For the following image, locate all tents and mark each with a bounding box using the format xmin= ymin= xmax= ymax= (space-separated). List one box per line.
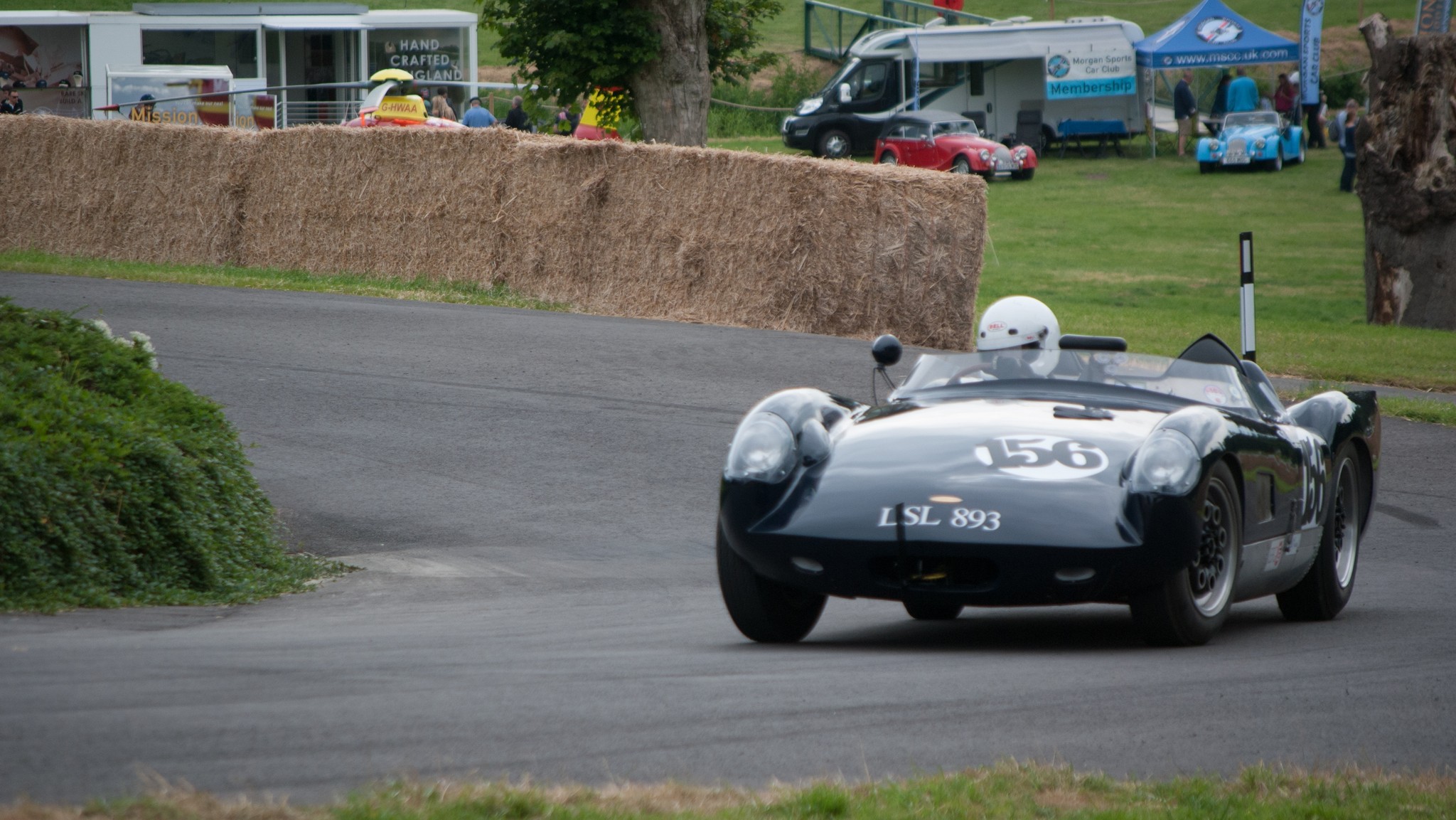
xmin=1131 ymin=0 xmax=1304 ymax=160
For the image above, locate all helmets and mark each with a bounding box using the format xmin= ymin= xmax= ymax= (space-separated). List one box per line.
xmin=976 ymin=296 xmax=1060 ymax=377
xmin=141 ymin=93 xmax=157 ymax=107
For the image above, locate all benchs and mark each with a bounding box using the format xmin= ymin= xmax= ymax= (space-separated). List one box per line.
xmin=1058 ymin=118 xmax=1129 ymax=159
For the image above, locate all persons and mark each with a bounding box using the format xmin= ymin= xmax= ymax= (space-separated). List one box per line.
xmin=418 ymin=87 xmax=591 ymax=136
xmin=1226 ymin=65 xmax=1261 ymax=113
xmin=1338 ymin=99 xmax=1359 ymax=156
xmin=1173 ymin=69 xmax=1197 ymax=159
xmin=0 ymin=84 xmax=23 ymax=115
xmin=1261 ymin=72 xmax=1294 ymax=120
xmin=1340 ymin=110 xmax=1360 ymax=195
xmin=128 ymin=93 xmax=162 ymax=123
xmin=970 ymin=295 xmax=1062 ymax=388
xmin=1216 ymin=73 xmax=1232 ymax=115
xmin=1288 ymin=67 xmax=1331 ymax=151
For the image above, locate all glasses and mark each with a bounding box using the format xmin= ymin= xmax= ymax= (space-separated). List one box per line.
xmin=9 ymin=95 xmax=18 ymax=99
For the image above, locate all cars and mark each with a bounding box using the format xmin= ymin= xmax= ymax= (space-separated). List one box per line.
xmin=875 ymin=109 xmax=1037 ymax=184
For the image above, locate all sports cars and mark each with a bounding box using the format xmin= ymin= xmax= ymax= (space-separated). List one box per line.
xmin=713 ymin=327 xmax=1382 ymax=652
xmin=1196 ymin=109 xmax=1307 ymax=173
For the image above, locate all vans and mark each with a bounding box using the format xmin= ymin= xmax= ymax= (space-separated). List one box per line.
xmin=775 ymin=13 xmax=1148 ymax=159
